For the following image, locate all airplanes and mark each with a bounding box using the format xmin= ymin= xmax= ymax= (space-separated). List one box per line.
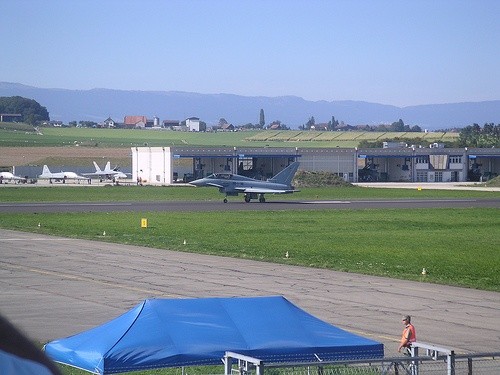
xmin=39 ymin=163 xmax=90 ymax=181
xmin=0 ymin=171 xmax=26 ymax=182
xmin=82 ymin=160 xmax=126 ymax=183
xmin=187 ymin=160 xmax=301 ymax=205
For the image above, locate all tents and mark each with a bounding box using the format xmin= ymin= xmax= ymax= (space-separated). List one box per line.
xmin=37 ymin=293 xmax=392 ymax=375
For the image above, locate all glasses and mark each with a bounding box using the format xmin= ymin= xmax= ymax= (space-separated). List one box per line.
xmin=402 ymin=319 xmax=408 ymax=321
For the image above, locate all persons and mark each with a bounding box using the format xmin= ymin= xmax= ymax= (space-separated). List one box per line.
xmin=397 ymin=315 xmax=416 ymax=358
